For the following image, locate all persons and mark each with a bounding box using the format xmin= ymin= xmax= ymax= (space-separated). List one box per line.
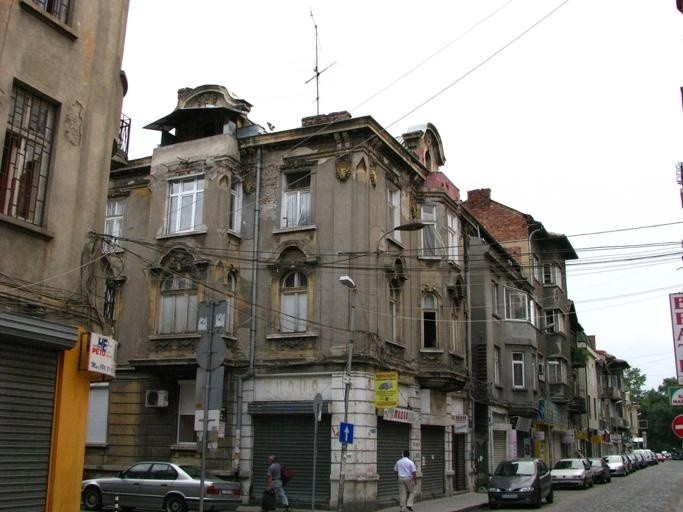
xmin=393 ymin=449 xmax=417 ymax=512
xmin=267 ymin=454 xmax=290 ymax=511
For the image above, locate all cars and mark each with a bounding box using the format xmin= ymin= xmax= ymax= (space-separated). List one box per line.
xmin=80 ymin=460 xmax=244 ymax=512
xmin=548 ymin=447 xmax=672 ymax=490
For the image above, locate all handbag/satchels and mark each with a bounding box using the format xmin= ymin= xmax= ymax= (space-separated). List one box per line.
xmin=281 ymin=467 xmax=294 ymax=486
xmin=262 ymin=489 xmax=277 ymax=511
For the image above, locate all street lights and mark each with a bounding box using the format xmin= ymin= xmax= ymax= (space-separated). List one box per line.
xmin=335 ymin=273 xmax=358 ymax=512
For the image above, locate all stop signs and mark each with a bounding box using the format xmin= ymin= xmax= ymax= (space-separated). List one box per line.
xmin=671 ymin=414 xmax=683 ymax=439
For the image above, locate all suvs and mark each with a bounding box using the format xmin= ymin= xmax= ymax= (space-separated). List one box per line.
xmin=487 ymin=456 xmax=557 ymax=509
